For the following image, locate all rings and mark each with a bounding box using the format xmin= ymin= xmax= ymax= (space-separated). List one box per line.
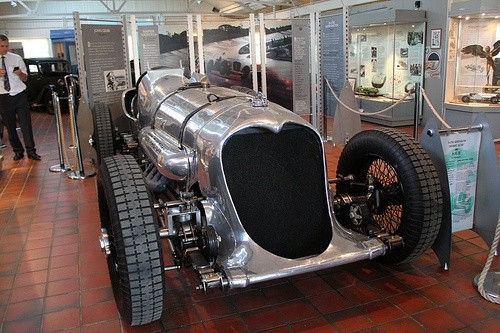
xmin=2 ymin=72 xmax=4 ymax=73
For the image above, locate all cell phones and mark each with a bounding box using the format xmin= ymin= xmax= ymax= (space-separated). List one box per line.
xmin=13 ymin=67 xmax=19 ymax=72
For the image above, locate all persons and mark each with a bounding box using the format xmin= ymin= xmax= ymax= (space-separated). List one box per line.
xmin=350 ymin=31 xmax=422 ymax=92
xmin=106 ymin=71 xmax=119 ymax=91
xmin=0 ymin=35 xmax=42 ymax=161
xmin=462 ymin=40 xmax=500 ymax=85
xmin=0 ymin=114 xmax=7 ymax=161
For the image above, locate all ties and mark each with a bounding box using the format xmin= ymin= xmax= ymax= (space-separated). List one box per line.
xmin=1 ymin=55 xmax=11 ymax=91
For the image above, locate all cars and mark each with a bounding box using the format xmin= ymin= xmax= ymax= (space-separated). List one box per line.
xmin=457 ymin=92 xmax=499 ymax=104
xmin=24 ymin=57 xmax=79 ymax=116
xmin=90 ymin=63 xmax=445 ymax=327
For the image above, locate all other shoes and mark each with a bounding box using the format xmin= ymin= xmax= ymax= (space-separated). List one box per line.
xmin=28 ymin=152 xmax=41 ymax=159
xmin=13 ymin=153 xmax=24 ymax=160
